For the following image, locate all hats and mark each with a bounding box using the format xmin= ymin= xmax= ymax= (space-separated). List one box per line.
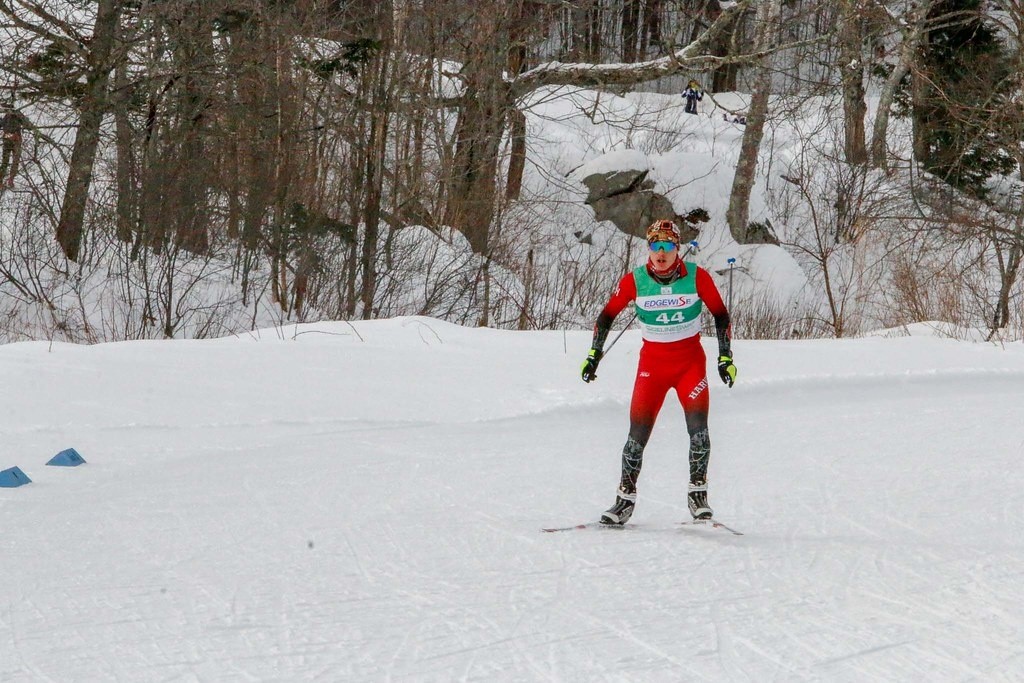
xmin=646 ymin=220 xmax=681 ymax=244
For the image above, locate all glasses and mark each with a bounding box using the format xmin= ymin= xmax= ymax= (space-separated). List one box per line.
xmin=651 ymin=241 xmax=674 ymax=251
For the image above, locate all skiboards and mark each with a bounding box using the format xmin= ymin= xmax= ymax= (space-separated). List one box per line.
xmin=540 ymin=517 xmax=744 ymax=537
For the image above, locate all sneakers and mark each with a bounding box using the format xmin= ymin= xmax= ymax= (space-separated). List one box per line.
xmin=601 ymin=495 xmax=635 ymax=525
xmin=688 ymin=489 xmax=713 ymax=519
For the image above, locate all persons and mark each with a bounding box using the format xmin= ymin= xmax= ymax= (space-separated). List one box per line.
xmin=682 ymin=79 xmax=704 ymax=114
xmin=0 ymin=104 xmax=33 ymax=187
xmin=580 ymin=220 xmax=737 ymax=527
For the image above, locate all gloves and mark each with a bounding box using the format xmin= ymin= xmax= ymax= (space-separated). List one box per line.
xmin=580 ymin=350 xmax=603 ymax=383
xmin=718 ymin=356 xmax=736 ymax=388
